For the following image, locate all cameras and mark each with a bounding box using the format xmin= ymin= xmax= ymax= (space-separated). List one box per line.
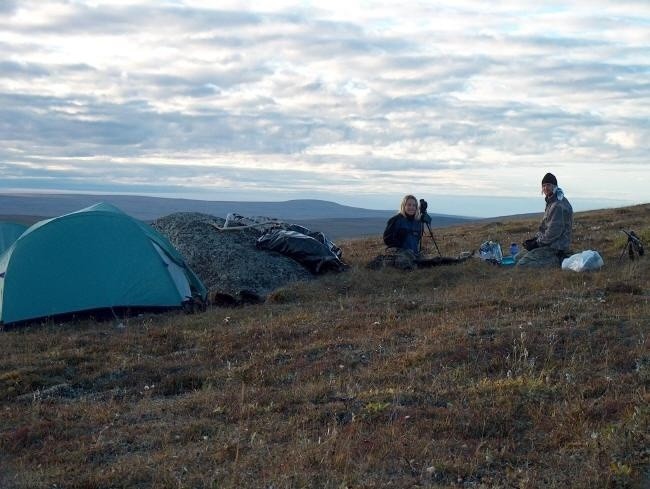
xmin=420 ymin=199 xmax=427 ymax=215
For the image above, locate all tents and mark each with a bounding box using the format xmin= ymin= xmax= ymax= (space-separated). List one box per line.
xmin=0 ymin=219 xmax=30 ymax=263
xmin=0 ymin=200 xmax=210 ymax=330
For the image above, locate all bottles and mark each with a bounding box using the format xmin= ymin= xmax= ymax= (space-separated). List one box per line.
xmin=498 ymin=257 xmax=518 ymax=265
xmin=510 ymin=243 xmax=518 ymax=258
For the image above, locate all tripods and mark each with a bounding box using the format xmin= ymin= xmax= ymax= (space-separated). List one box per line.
xmin=419 ymin=215 xmax=441 ymax=256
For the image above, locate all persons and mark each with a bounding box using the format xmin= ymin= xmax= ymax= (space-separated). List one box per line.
xmin=512 ymin=172 xmax=573 ymax=267
xmin=381 ymin=194 xmax=425 ymax=270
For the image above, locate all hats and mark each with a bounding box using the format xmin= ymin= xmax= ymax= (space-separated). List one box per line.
xmin=542 ymin=173 xmax=557 ymax=185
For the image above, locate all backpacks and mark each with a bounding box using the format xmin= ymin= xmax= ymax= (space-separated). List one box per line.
xmin=477 ymin=241 xmax=502 ymax=264
